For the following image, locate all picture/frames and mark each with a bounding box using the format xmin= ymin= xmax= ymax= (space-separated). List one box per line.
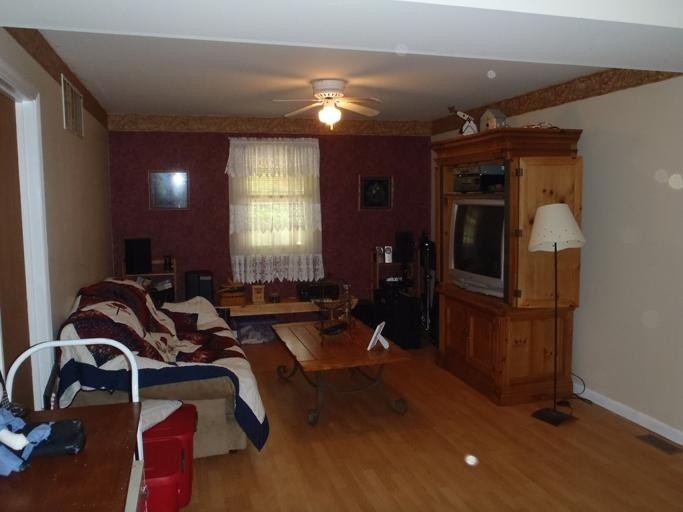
xmin=147 ymin=166 xmax=191 ymax=212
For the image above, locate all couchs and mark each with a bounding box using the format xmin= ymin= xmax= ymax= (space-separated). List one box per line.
xmin=55 ymin=276 xmax=252 ymax=459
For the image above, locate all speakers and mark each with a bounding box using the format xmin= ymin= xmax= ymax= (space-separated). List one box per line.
xmin=125 ymin=238 xmax=153 ymax=273
xmin=395 ymin=231 xmax=414 ymax=262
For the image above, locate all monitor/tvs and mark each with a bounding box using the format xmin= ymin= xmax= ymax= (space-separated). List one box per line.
xmin=448 ymin=198 xmax=505 ymax=299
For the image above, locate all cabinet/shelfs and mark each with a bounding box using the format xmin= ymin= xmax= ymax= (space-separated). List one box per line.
xmin=429 ymin=127 xmax=584 ymax=406
xmin=0 ymin=337 xmax=147 ymax=512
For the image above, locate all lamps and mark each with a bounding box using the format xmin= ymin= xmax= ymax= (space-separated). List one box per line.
xmin=318 ymin=98 xmax=341 ymax=125
xmin=527 ymin=203 xmax=589 ymax=426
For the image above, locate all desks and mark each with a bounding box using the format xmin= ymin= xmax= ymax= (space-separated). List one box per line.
xmin=229 ymin=293 xmax=358 ymax=346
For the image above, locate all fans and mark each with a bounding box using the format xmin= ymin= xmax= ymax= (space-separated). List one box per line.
xmin=270 ymin=79 xmax=384 ymax=119
